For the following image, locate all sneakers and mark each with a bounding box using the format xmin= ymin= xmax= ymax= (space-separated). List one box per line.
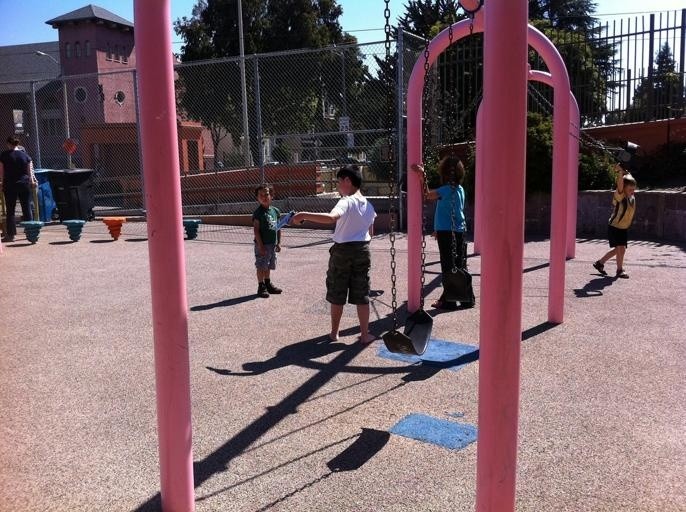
xmin=258 ymin=283 xmax=282 ymax=297
xmin=617 ymin=270 xmax=629 ymax=278
xmin=593 ymin=260 xmax=608 ymax=275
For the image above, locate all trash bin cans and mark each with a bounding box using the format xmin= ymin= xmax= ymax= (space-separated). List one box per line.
xmin=28 ymin=169 xmax=97 ymax=222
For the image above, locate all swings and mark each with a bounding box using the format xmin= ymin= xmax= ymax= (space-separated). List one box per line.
xmin=445 ymin=0 xmax=485 ymax=304
xmin=528 ymin=83 xmax=640 ymax=164
xmin=382 ymin=0 xmax=433 ymax=356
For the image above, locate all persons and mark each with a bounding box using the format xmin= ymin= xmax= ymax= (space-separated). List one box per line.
xmin=252 ymin=184 xmax=283 ymax=298
xmin=593 ymin=162 xmax=636 ymax=279
xmin=0 ymin=137 xmax=39 ymax=242
xmin=289 ymin=165 xmax=377 ymax=343
xmin=411 ymin=157 xmax=476 ymax=309
